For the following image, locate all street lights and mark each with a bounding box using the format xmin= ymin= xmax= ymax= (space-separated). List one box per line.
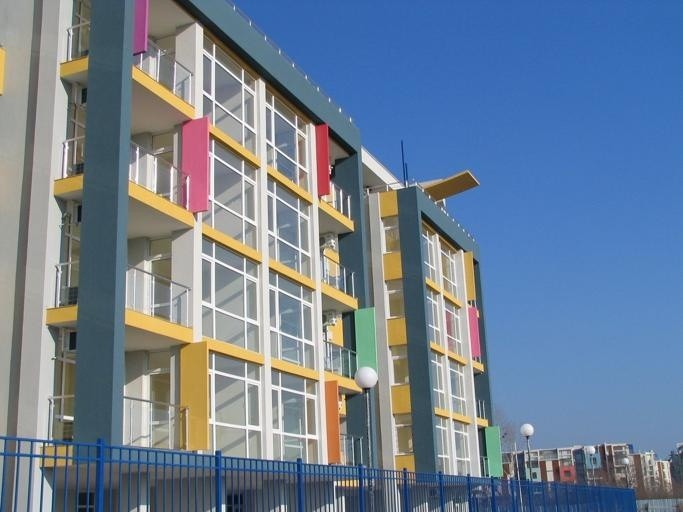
xmin=584 ymin=445 xmax=597 ymax=512
xmin=354 ymin=365 xmax=378 ymax=512
xmin=521 ymin=423 xmax=534 ymax=511
xmin=621 ymin=458 xmax=630 ymax=512
